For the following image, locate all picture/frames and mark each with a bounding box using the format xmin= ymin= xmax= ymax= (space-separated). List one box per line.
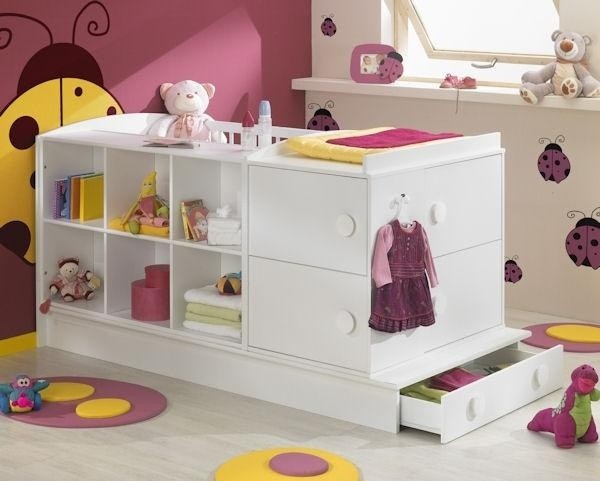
xmin=349 ymin=44 xmax=402 ymax=84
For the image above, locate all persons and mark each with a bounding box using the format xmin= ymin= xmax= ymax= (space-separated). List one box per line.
xmin=362 ymin=54 xmax=375 ymax=72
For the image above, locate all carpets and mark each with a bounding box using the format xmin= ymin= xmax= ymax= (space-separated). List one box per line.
xmin=522 ymin=322 xmax=599 ymax=353
xmin=217 ymin=448 xmax=362 ymax=481
xmin=2 ymin=377 xmax=168 ymax=429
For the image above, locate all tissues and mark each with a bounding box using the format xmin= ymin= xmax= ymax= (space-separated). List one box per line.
xmin=204 ymin=204 xmax=242 ymax=245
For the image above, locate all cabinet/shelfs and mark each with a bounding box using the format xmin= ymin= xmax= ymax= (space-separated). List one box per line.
xmin=375 ymin=148 xmax=506 ymax=261
xmin=370 ymin=240 xmax=507 ymax=371
xmin=248 ymin=161 xmax=372 ymax=273
xmin=34 ymin=113 xmax=248 ymax=393
xmin=368 ymin=328 xmax=565 ymax=443
xmin=249 ymin=256 xmax=370 ymax=421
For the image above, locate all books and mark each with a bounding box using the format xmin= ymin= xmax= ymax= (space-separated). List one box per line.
xmin=179 ymin=198 xmax=209 ymax=245
xmin=49 ymin=169 xmax=104 ymax=224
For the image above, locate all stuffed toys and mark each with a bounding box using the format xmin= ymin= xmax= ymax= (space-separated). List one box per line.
xmin=526 ymin=362 xmax=599 ymax=451
xmin=37 ymin=256 xmax=101 ymax=316
xmin=152 ymin=80 xmax=227 ymax=143
xmin=519 ymin=28 xmax=599 ymax=106
xmin=1 ymin=374 xmax=50 ymax=417
xmin=120 ymin=168 xmax=171 ymax=236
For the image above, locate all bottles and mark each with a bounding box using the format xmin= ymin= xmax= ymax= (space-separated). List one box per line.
xmin=241 ymin=99 xmax=273 ymax=151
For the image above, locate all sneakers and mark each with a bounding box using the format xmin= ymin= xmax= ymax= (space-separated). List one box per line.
xmin=458 ymin=77 xmax=476 ymax=89
xmin=439 ymin=73 xmax=458 ymax=88
